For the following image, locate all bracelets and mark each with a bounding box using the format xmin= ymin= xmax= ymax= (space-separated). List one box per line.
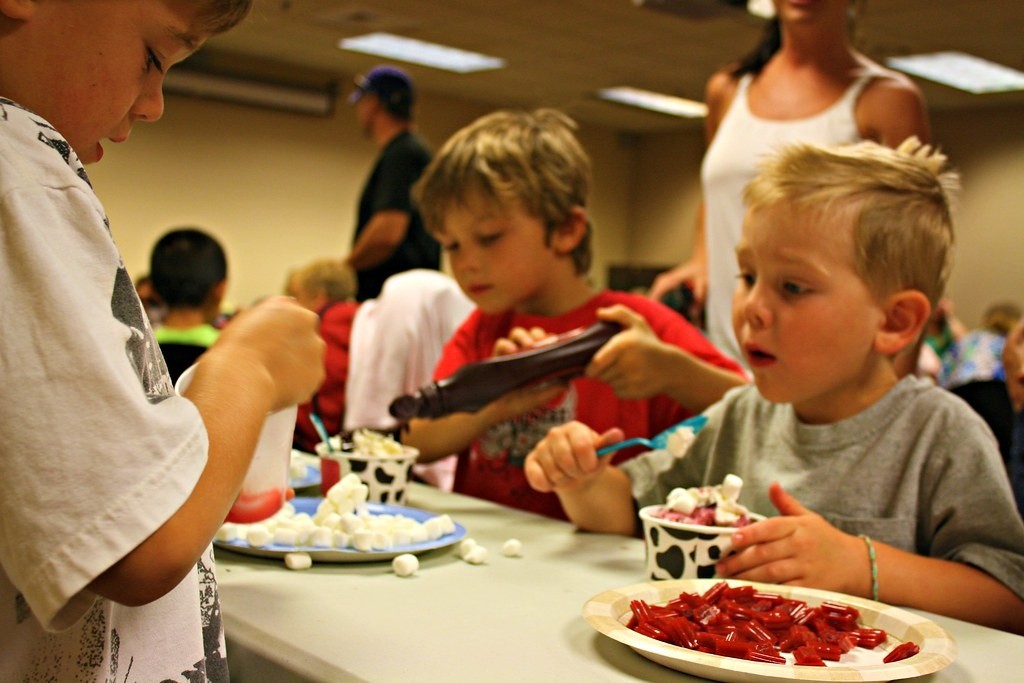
xmin=857 ymin=534 xmax=878 ymax=601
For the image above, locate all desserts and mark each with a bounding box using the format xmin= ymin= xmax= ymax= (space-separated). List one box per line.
xmin=493 ymin=326 xmax=546 ymax=355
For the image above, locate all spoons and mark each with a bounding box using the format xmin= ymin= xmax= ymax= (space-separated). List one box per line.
xmin=596 ymin=414 xmax=708 ymax=455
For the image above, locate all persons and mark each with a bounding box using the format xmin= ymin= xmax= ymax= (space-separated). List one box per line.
xmin=527 ymin=137 xmax=1024 ymax=635
xmin=650 ymin=0 xmax=929 ymax=377
xmin=334 ymin=68 xmax=445 ymax=303
xmin=279 ymin=267 xmax=361 ymax=454
xmin=400 ymin=107 xmax=745 ymax=520
xmin=143 ymin=227 xmax=229 ymax=393
xmin=1 ymin=0 xmax=325 ymax=683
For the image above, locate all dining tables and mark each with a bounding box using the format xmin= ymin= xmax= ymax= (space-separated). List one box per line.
xmin=214 ymin=477 xmax=1024 ymax=681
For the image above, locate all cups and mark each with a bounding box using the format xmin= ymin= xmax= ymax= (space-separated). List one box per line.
xmin=174 ymin=360 xmax=297 ymax=524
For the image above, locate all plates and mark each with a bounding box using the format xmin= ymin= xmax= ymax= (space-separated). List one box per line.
xmin=212 ymin=497 xmax=467 ymax=563
xmin=289 ymin=465 xmax=321 ymax=494
xmin=580 ymin=578 xmax=957 ymax=682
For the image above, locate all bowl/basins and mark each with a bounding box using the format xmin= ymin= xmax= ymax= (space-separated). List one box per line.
xmin=314 ymin=442 xmax=419 ymax=505
xmin=639 ymin=505 xmax=768 ymax=580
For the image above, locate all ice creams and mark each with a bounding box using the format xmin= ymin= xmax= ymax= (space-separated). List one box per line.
xmin=327 ymin=428 xmax=401 ymax=456
xmin=648 ymin=473 xmax=761 ymax=526
